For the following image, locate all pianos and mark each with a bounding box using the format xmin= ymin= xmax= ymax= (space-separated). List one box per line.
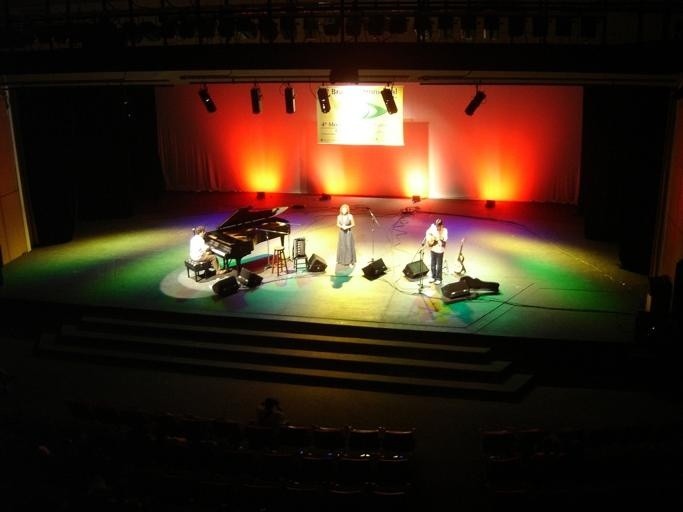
xmin=202 ymin=206 xmax=290 ymax=277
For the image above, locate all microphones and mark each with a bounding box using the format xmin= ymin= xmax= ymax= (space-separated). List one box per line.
xmin=421 ymin=237 xmax=426 ymax=246
xmin=368 ymin=208 xmax=373 ymax=216
xmin=191 ymin=227 xmax=195 ymax=235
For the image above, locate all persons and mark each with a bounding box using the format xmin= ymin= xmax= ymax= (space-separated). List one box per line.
xmin=255 ymin=397 xmax=287 ymax=427
xmin=188 ymin=226 xmax=226 ymax=276
xmin=425 ymin=218 xmax=448 ymax=285
xmin=335 ymin=204 xmax=355 ymax=266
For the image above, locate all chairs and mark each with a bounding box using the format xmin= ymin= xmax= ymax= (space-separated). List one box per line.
xmin=293 ymin=244 xmax=309 ymax=272
xmin=169 ymin=411 xmax=425 ymax=464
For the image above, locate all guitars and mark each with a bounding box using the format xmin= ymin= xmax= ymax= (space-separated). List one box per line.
xmin=428 ymin=233 xmax=444 ymax=247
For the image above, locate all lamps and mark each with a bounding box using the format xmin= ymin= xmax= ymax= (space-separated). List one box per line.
xmin=193 ymin=76 xmax=488 ymax=117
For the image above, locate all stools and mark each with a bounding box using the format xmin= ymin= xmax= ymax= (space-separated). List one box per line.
xmin=272 ymin=247 xmax=289 ymax=275
xmin=185 ymin=257 xmax=220 ymax=283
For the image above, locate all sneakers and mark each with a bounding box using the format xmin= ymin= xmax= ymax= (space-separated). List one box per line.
xmin=428 ymin=278 xmax=441 ymax=285
xmin=215 ymin=270 xmax=227 ymax=275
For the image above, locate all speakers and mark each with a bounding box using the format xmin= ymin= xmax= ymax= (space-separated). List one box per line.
xmin=441 ymin=280 xmax=470 ymax=298
xmin=308 ymin=253 xmax=327 ymax=272
xmin=212 ymin=276 xmax=240 ymax=295
xmin=361 ymin=257 xmax=388 ymax=277
xmin=237 ymin=266 xmax=263 ymax=286
xmin=402 ymin=260 xmax=429 ymax=279
